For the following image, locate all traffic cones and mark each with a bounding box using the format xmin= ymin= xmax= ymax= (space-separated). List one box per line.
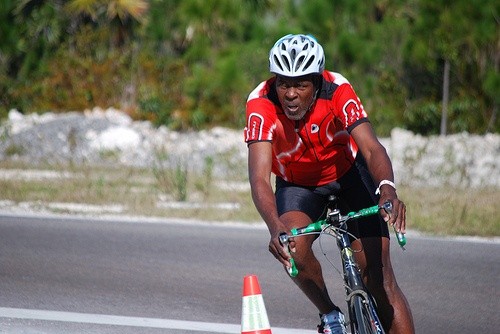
xmin=241 ymin=274 xmax=273 ymax=334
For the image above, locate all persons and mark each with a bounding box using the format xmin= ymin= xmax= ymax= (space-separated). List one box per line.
xmin=245 ymin=33 xmax=415 ymax=333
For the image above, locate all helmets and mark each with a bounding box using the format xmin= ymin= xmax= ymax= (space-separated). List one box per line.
xmin=269 ymin=33 xmax=326 ymax=77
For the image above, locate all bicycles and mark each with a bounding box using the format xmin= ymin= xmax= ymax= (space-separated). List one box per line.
xmin=277 ymin=196 xmax=407 ymax=334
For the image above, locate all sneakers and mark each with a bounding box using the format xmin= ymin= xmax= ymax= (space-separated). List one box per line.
xmin=321 ymin=307 xmax=347 ymax=334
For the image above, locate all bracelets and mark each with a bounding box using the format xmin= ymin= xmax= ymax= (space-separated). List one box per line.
xmin=375 ymin=179 xmax=396 ymax=194
